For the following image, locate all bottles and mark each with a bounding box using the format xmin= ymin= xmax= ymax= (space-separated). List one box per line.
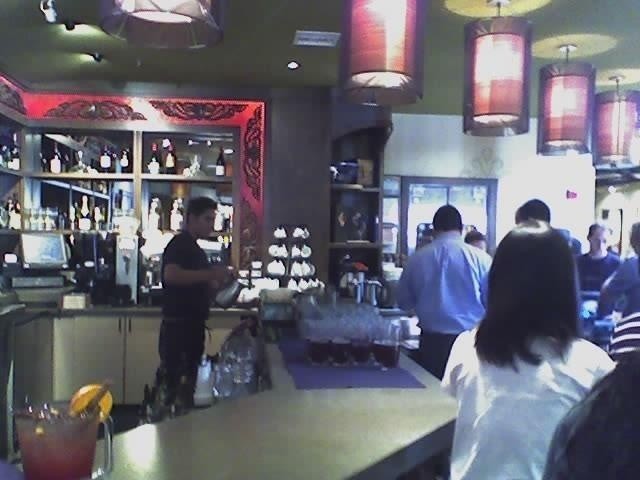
xmin=0 ymin=142 xmax=230 ymax=231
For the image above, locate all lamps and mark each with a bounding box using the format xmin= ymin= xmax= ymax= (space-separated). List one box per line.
xmin=335 ymin=0 xmax=423 ymax=107
xmin=96 ymin=0 xmax=223 ymax=51
xmin=460 ymin=0 xmax=640 ymax=169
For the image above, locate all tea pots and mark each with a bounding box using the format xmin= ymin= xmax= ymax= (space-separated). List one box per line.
xmin=215 ymin=279 xmax=252 ymax=311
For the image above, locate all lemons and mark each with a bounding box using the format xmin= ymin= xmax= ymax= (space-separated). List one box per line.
xmin=69 ymin=383 xmax=114 ymax=422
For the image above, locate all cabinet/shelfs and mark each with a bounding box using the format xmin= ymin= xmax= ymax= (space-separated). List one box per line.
xmin=327 ymin=86 xmax=393 ymax=289
xmin=8 ymin=315 xmax=246 ymax=409
xmin=0 ymin=71 xmax=266 ymax=303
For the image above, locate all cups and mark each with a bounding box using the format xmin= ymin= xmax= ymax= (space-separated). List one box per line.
xmin=14 ymin=398 xmax=116 ymax=480
xmin=298 ymin=314 xmax=401 ymax=371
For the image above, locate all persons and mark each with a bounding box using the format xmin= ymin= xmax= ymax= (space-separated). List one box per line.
xmin=540 ymin=351 xmax=640 ymax=479
xmin=515 ymin=201 xmax=584 ymax=257
xmin=606 ymin=283 xmax=640 ymax=368
xmin=594 ymin=220 xmax=640 ymax=317
xmin=462 ymin=230 xmax=487 ymax=252
xmin=419 ymin=229 xmax=434 ymax=245
xmin=572 ymin=221 xmax=625 ymax=352
xmin=396 ymin=203 xmax=494 ymax=382
xmin=441 ymin=218 xmax=616 ymax=479
xmin=156 ymin=195 xmax=244 ymax=420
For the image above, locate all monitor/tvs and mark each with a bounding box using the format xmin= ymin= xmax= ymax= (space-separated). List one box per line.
xmin=20 ymin=231 xmax=70 ymax=276
